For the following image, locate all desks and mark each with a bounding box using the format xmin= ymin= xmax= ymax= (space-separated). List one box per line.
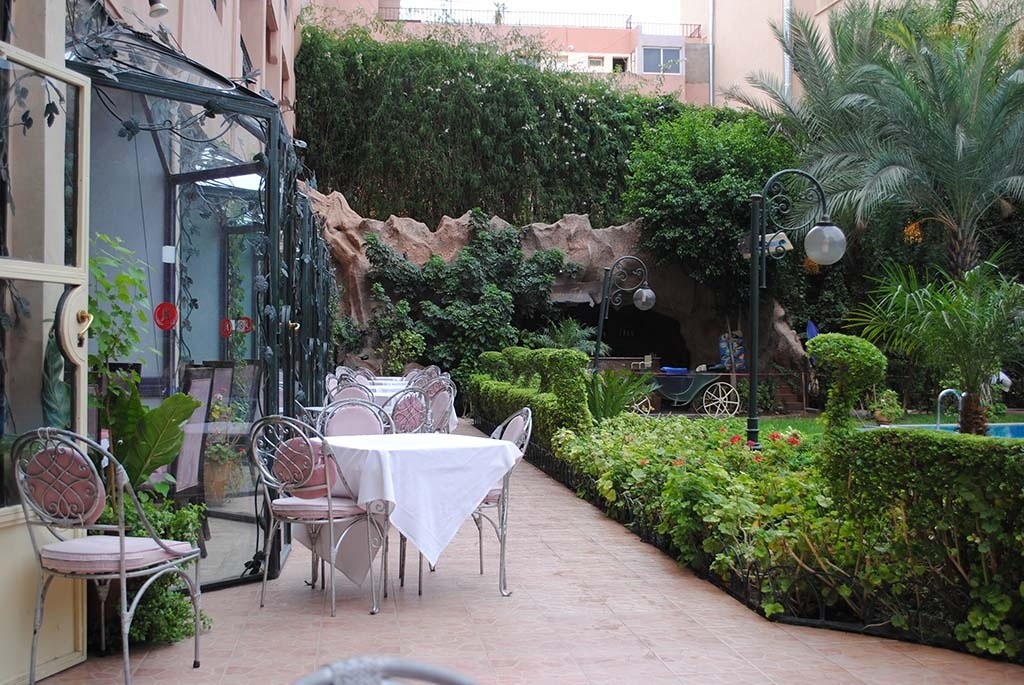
xmin=302 ymin=406 xmax=394 ymax=428
xmin=306 ymin=434 xmax=521 ymax=614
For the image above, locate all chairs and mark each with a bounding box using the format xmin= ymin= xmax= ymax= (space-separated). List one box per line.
xmin=10 ymin=427 xmax=202 ymax=685
xmin=248 ymin=362 xmax=532 ymax=619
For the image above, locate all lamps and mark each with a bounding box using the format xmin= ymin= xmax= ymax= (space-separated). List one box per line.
xmin=147 ymin=0 xmax=168 ymax=18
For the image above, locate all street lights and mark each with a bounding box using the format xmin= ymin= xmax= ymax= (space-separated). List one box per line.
xmin=747 ymin=169 xmax=847 ymax=450
xmin=593 ymin=256 xmax=657 ymax=369
